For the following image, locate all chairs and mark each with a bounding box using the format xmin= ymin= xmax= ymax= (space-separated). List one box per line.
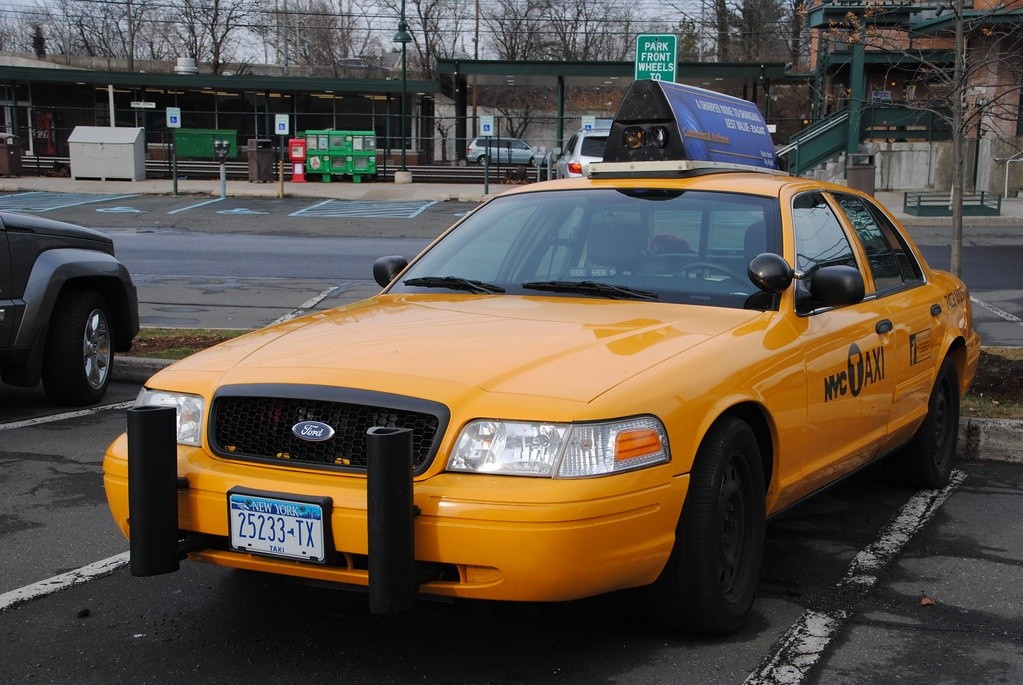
xmin=722 ymin=216 xmax=804 ymax=294
xmin=568 ymin=220 xmax=647 ymax=288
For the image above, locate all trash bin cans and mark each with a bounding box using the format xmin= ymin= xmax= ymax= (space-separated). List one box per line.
xmin=33 ymin=138 xmax=48 ymax=156
xmin=0 ymin=133 xmax=23 ymax=177
xmin=247 ymin=139 xmax=273 ymax=182
xmin=845 ymin=151 xmax=876 ymax=196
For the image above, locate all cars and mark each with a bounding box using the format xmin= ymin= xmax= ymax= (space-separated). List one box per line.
xmin=553 ymin=126 xmax=610 ymax=180
xmin=103 ymin=80 xmax=982 ymax=646
xmin=1 ymin=211 xmax=141 ymax=409
xmin=465 ymin=138 xmax=536 ymax=168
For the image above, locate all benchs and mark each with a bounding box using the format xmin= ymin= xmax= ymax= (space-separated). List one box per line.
xmin=650 ymin=254 xmax=746 ymax=290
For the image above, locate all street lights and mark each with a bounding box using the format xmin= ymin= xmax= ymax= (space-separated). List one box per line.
xmin=394 ymin=0 xmax=416 ymax=183
